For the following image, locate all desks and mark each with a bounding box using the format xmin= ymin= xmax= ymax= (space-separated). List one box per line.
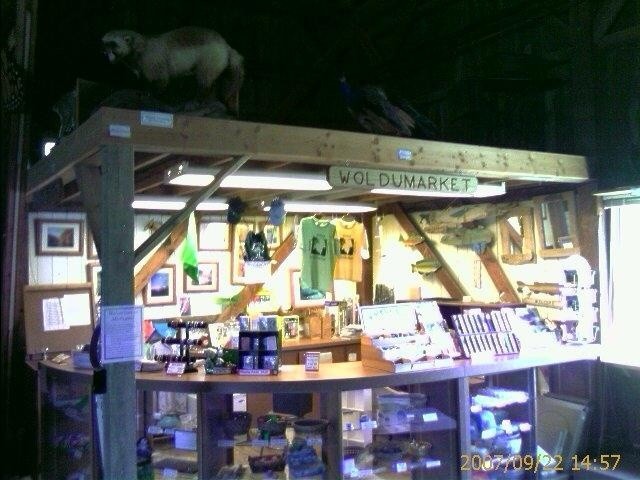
xmin=396 ymin=298 xmax=526 ymax=330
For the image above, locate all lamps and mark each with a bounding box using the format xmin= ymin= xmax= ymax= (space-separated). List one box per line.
xmin=369 ymin=180 xmax=507 ymax=199
xmin=163 ymin=164 xmax=334 ymax=192
xmin=133 ymin=191 xmax=232 ymax=215
xmin=257 ymin=197 xmax=380 ymax=215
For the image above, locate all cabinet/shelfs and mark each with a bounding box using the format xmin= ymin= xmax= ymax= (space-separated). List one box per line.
xmin=38 ymin=333 xmax=599 ymax=480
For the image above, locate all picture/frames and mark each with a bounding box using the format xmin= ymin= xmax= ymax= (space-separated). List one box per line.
xmin=231 ymin=221 xmax=256 ymax=287
xmin=288 ymin=266 xmax=327 ymax=310
xmin=139 ymin=262 xmax=178 ymax=308
xmin=87 ymin=227 xmax=100 ymax=261
xmin=86 ymin=261 xmax=104 ymax=332
xmin=195 ymin=217 xmax=233 ymax=252
xmin=181 ymin=260 xmax=220 ymax=294
xmin=258 ymin=220 xmax=282 ymax=252
xmin=36 ymin=215 xmax=86 ymax=258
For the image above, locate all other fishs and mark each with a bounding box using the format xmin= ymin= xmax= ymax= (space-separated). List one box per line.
xmin=411 ymin=259 xmax=442 ymax=277
xmin=398 ymin=233 xmax=426 ymax=248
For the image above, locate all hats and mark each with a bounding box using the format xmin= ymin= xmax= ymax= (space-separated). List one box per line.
xmin=227 ymin=197 xmax=244 ymax=224
xmin=269 ymin=200 xmax=286 ymax=225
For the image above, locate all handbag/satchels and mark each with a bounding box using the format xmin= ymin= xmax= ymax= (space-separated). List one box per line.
xmin=244 ymin=231 xmax=270 ymax=260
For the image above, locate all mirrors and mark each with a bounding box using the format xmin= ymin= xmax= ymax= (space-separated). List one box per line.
xmin=500 ymin=191 xmax=580 ymax=263
